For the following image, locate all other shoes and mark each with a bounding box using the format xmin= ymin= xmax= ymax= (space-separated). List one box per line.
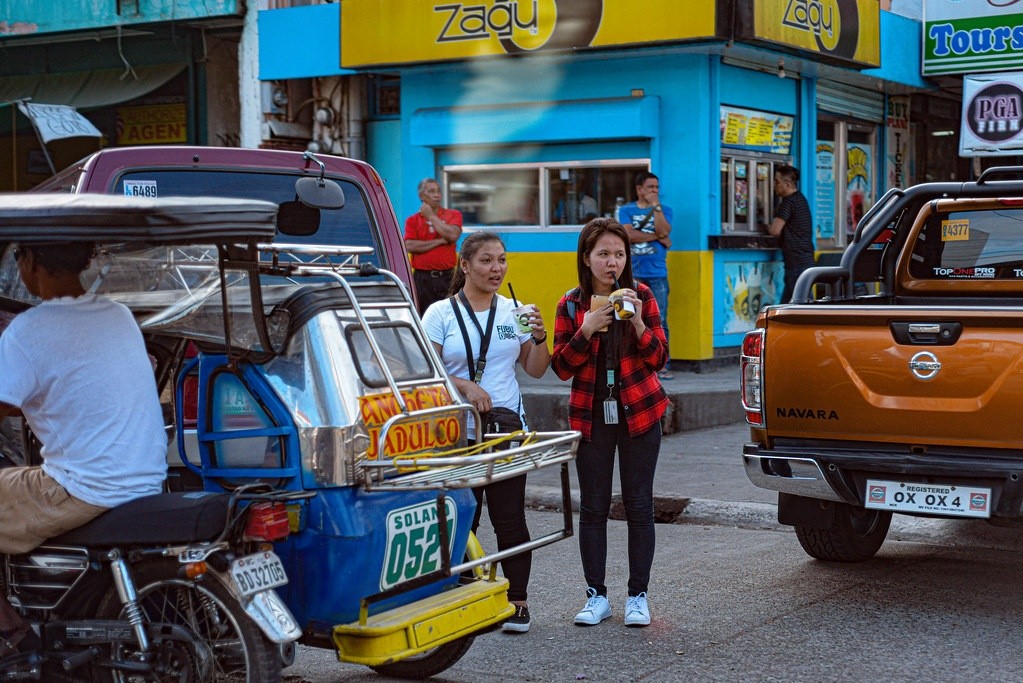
xmin=0 ymin=630 xmax=48 ymax=668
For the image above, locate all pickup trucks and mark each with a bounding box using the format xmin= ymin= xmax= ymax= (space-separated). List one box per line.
xmin=738 ymin=166 xmax=1023 ymax=564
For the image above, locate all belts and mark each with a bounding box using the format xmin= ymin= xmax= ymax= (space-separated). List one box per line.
xmin=415 ymin=269 xmax=452 ymax=279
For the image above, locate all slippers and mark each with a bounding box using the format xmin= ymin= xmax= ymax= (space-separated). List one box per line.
xmin=657 ymin=370 xmax=674 ymax=380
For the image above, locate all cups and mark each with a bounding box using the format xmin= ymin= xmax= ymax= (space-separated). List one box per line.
xmin=609 ymin=288 xmax=636 ymax=320
xmin=511 ymin=304 xmax=536 ymax=333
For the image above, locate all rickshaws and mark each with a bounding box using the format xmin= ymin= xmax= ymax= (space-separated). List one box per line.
xmin=1 ymin=191 xmax=587 ymax=683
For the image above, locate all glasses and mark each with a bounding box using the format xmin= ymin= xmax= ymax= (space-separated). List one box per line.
xmin=13 ymin=247 xmax=27 ymax=261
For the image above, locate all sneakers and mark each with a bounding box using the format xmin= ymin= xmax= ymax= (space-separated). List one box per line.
xmin=574 ymin=587 xmax=612 ymax=624
xmin=624 ymin=591 xmax=650 ymax=626
xmin=502 ymin=604 xmax=531 ymax=632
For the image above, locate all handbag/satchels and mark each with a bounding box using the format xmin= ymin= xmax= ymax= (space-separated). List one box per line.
xmin=480 ymin=407 xmax=523 ymax=450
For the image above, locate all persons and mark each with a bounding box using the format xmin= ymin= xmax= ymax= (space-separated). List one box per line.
xmin=0 ymin=242 xmax=164 ymax=674
xmin=765 ymin=175 xmax=816 ymax=302
xmin=553 ymin=174 xmax=599 ymax=224
xmin=419 ymin=230 xmax=550 ymax=634
xmin=619 ymin=171 xmax=674 ymax=380
xmin=552 ymin=218 xmax=671 ymax=628
xmin=404 ymin=178 xmax=462 ymax=322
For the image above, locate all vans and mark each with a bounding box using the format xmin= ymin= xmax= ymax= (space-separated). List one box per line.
xmin=0 ymin=145 xmax=417 ymax=315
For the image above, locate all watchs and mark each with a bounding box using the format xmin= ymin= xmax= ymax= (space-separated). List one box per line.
xmin=530 ymin=330 xmax=547 ymax=345
xmin=655 ymin=206 xmax=661 ymax=213
